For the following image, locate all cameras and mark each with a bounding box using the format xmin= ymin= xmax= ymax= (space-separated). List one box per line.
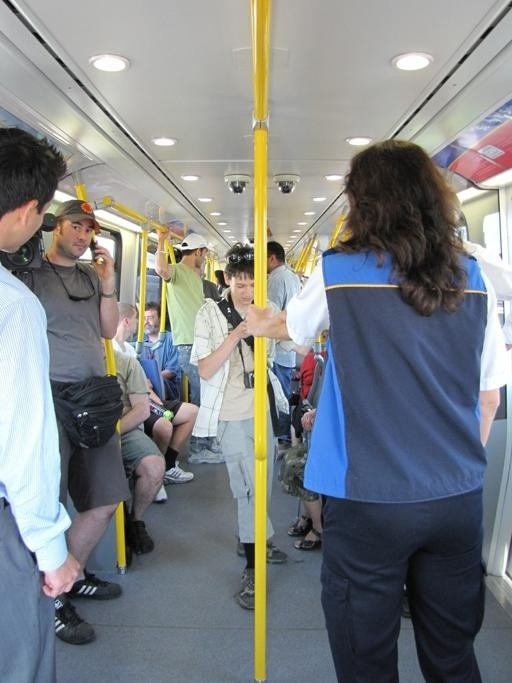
xmin=244 ymin=369 xmax=270 ymax=389
xmin=0 ymin=212 xmax=58 ymax=271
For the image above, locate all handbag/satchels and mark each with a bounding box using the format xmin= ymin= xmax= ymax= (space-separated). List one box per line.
xmin=215 ymin=298 xmax=291 ymax=436
xmin=55 ymin=373 xmax=125 ymax=450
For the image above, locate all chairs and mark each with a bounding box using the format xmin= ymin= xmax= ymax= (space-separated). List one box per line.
xmin=137 ymin=359 xmax=166 ymax=402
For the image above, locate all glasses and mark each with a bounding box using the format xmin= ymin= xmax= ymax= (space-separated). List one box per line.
xmin=59 ymin=271 xmax=97 ymax=301
xmin=226 ymin=252 xmax=254 ymax=264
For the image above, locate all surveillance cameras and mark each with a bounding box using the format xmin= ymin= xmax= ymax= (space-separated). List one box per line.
xmin=223 ymin=174 xmax=251 ymax=195
xmin=272 ymin=175 xmax=301 ymax=194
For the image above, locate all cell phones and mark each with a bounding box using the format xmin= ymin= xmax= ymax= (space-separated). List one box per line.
xmin=89 ymin=238 xmax=99 ymax=263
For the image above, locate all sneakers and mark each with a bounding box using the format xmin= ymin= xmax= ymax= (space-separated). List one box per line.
xmin=237 ymin=563 xmax=256 ymax=609
xmin=165 ymin=466 xmax=194 ymax=484
xmin=133 ymin=521 xmax=155 ymax=552
xmin=72 ymin=572 xmax=122 ymax=598
xmin=54 ymin=598 xmax=95 ymax=645
xmin=153 ymin=483 xmax=167 ymax=503
xmin=236 ymin=540 xmax=288 ymax=563
xmin=188 ymin=448 xmax=224 ymax=464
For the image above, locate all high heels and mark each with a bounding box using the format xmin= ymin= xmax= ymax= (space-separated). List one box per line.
xmin=294 ymin=528 xmax=321 ymax=550
xmin=288 ymin=515 xmax=312 ymax=536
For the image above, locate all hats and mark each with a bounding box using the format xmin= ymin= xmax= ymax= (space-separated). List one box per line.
xmin=55 ymin=200 xmax=100 ymax=231
xmin=181 ymin=232 xmax=206 ymax=250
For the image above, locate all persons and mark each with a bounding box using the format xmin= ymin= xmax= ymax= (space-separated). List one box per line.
xmin=190 ymin=244 xmax=290 ymax=609
xmin=24 ymin=200 xmax=131 ymax=645
xmin=153 ymin=225 xmax=228 ymax=466
xmin=1 ymin=127 xmax=85 ymax=682
xmin=285 ymin=139 xmax=512 ymax=682
xmin=288 ymin=329 xmax=331 ymax=446
xmin=279 ymin=408 xmax=348 ymax=551
xmin=212 ymin=270 xmax=230 ymax=296
xmin=111 ymin=302 xmax=196 ymax=504
xmin=138 ymin=302 xmax=182 ymax=400
xmin=113 ymin=348 xmax=166 ymax=568
xmin=267 ymin=241 xmax=301 ymax=400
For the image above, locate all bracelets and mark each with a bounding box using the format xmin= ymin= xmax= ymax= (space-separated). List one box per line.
xmin=153 ymin=251 xmax=166 ymax=255
xmin=100 ymin=289 xmax=118 ymax=300
